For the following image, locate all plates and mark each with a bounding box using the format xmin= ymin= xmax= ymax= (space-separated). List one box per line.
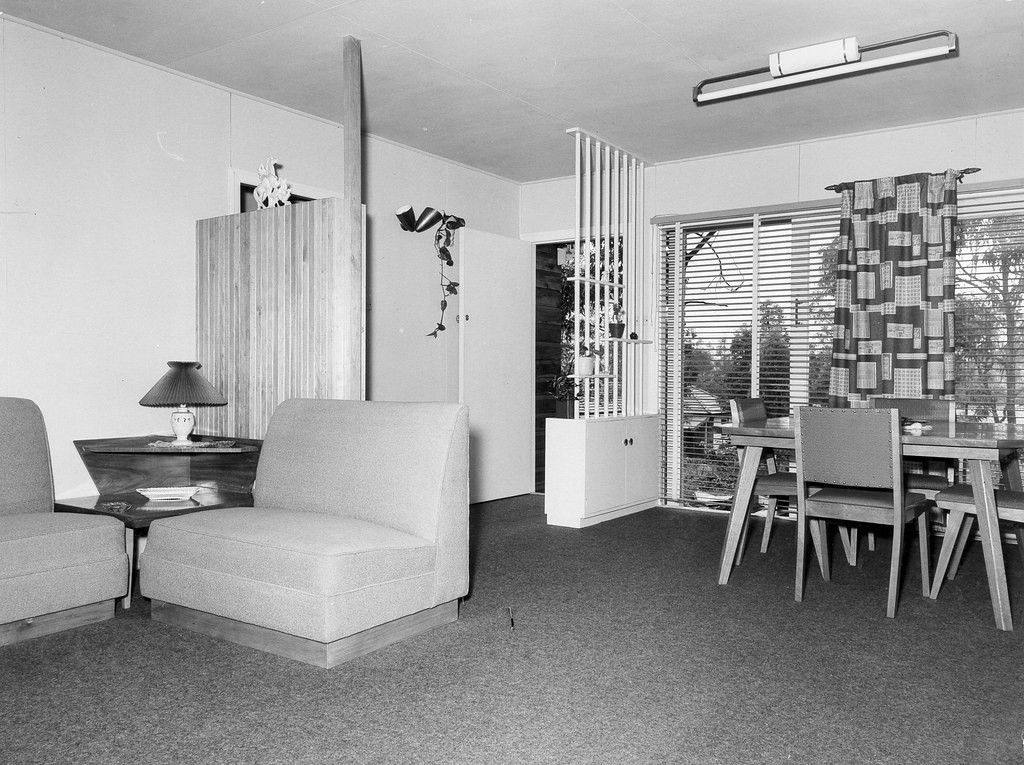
xmin=136 ymin=487 xmax=201 ymax=502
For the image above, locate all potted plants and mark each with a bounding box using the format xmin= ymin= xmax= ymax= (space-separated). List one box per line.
xmin=608 ymin=302 xmax=625 ymax=339
xmin=578 ymin=341 xmax=604 ymax=375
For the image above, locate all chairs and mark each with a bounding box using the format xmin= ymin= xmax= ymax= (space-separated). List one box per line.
xmin=727 ymin=398 xmax=1024 ymax=620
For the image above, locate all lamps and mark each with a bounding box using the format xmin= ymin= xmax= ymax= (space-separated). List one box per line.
xmin=138 ymin=362 xmax=230 ymax=446
xmin=396 ymin=205 xmax=442 ymax=233
xmin=692 ymin=29 xmax=959 ymax=103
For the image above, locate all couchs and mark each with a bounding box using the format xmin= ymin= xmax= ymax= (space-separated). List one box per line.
xmin=139 ymin=398 xmax=469 ymax=670
xmin=0 ymin=397 xmax=129 ymax=646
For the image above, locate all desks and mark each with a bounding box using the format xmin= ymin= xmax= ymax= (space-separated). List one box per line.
xmin=71 ymin=436 xmax=260 ymax=493
xmin=717 ymin=417 xmax=1024 ymax=631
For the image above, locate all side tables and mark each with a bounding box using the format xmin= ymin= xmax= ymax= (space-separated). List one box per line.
xmin=54 ymin=484 xmax=254 ymax=609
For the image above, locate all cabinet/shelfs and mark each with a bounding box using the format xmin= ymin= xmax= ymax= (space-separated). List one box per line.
xmin=542 ymin=414 xmax=660 ymax=529
